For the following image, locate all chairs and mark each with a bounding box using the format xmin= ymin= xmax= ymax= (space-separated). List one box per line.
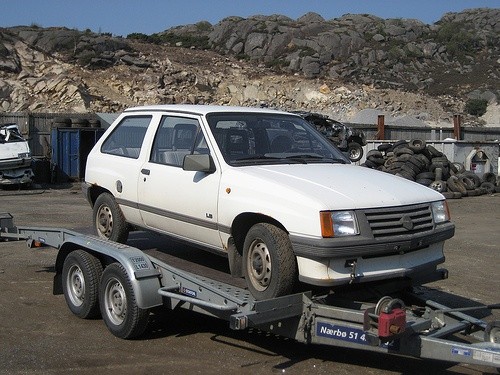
xmin=160 ymin=122 xmax=296 ymax=173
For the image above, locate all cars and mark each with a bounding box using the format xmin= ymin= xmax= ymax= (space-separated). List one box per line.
xmin=230 ymin=111 xmax=367 ymax=162
xmin=84 ymin=104 xmax=456 ymax=302
xmin=0 ymin=123 xmax=39 ymax=189
